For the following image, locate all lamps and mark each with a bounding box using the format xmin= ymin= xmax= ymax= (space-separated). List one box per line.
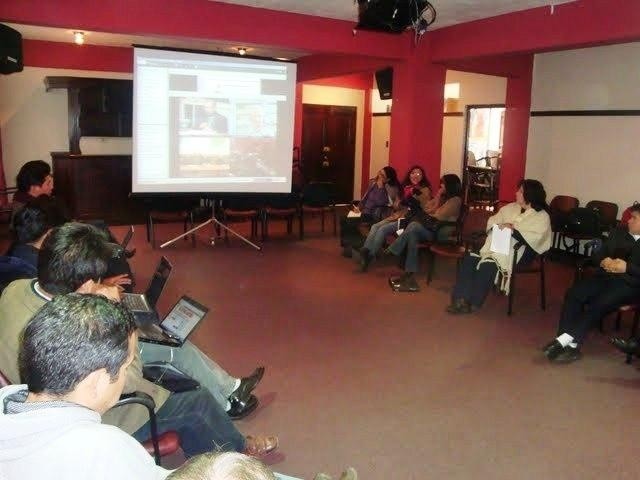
xmin=238 ymin=48 xmax=246 ymax=55
xmin=73 ymin=31 xmax=84 ymax=45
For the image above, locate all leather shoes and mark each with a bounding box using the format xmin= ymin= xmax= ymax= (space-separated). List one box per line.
xmin=245 ymin=435 xmax=279 ymax=458
xmin=610 ymin=337 xmax=639 ymax=354
xmin=226 ymin=394 xmax=259 ymax=419
xmin=228 ymin=367 xmax=265 ymax=413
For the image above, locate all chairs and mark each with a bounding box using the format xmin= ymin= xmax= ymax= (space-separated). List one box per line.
xmin=0 ymin=187 xmax=18 ymax=255
xmin=298 ymin=181 xmax=341 ymax=237
xmin=143 ymin=192 xmax=201 ymax=248
xmin=467 ymin=151 xmax=499 ymax=201
xmin=258 ymin=192 xmax=304 ymax=240
xmin=485 ymin=200 xmax=548 ymax=316
xmin=578 ymin=238 xmax=640 ymax=332
xmin=0 ymin=370 xmax=182 ymax=465
xmin=340 ymin=198 xmax=491 ymax=287
xmin=548 ymin=195 xmax=619 ymax=259
xmin=216 ymin=192 xmax=258 ymax=243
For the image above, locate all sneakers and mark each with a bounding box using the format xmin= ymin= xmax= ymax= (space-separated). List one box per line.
xmin=313 ymin=472 xmax=331 ymax=480
xmin=542 ymin=340 xmax=563 ymax=360
xmin=556 ymin=345 xmax=579 ymax=360
xmin=447 ymin=303 xmax=479 ymax=313
xmin=339 ymin=467 xmax=357 ymax=479
xmin=353 ymin=263 xmax=367 ymax=273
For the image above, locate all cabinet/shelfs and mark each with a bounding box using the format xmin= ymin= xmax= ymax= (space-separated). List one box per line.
xmin=44 ymin=76 xmax=132 ymax=155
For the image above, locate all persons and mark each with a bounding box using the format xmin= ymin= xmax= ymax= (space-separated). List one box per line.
xmin=165 ymin=449 xmax=276 ymax=480
xmin=0 ymin=193 xmax=265 ymax=421
xmin=351 ymin=167 xmax=432 ymax=275
xmin=443 ymin=178 xmax=554 ymax=314
xmin=7 ymin=159 xmax=137 ymax=258
xmin=607 ymin=328 xmax=640 ymax=360
xmin=193 ymin=100 xmax=227 ymax=132
xmin=0 ymin=221 xmax=281 ymax=463
xmin=540 ymin=201 xmax=640 ymax=364
xmin=0 ymin=293 xmax=358 ymax=480
xmin=383 ymin=174 xmax=464 ymax=284
xmin=341 ymin=165 xmax=401 ymax=259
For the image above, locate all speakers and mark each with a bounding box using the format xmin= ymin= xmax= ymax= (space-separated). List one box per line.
xmin=374 ymin=66 xmax=394 ymax=101
xmin=0 ymin=25 xmax=25 ymax=76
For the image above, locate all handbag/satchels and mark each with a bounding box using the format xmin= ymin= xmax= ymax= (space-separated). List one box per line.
xmin=400 ymin=205 xmax=440 ymax=236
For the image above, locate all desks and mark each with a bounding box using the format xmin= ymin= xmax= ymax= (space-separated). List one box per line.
xmin=49 ymin=151 xmax=144 ymax=226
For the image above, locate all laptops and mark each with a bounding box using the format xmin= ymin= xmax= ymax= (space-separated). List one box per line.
xmin=139 ymin=296 xmax=211 ymax=348
xmin=117 ymin=224 xmax=134 ymax=249
xmin=123 ymin=255 xmax=174 ymax=311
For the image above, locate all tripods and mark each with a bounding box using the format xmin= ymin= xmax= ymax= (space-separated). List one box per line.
xmin=158 ymin=201 xmax=262 ymax=253
